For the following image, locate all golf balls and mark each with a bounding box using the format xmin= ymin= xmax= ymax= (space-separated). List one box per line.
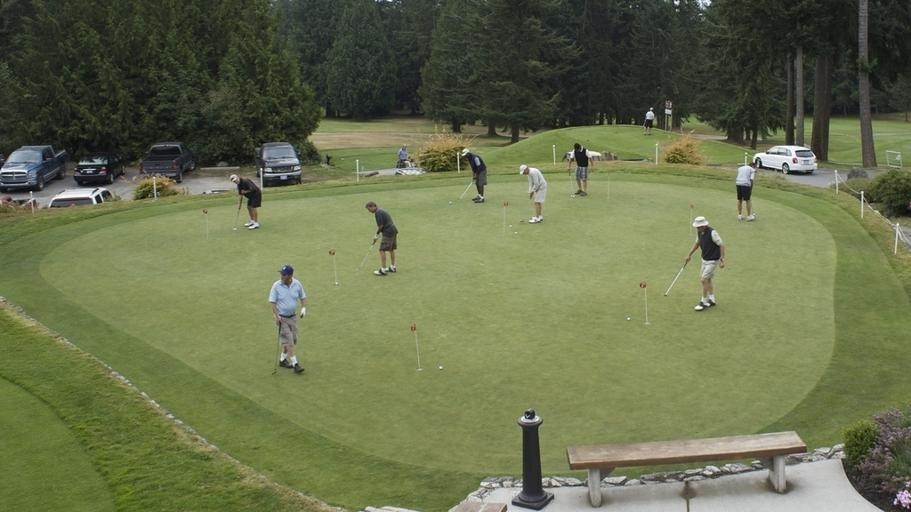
xmin=439 ymin=365 xmax=443 ymax=370
xmin=448 ymin=201 xmax=453 ymax=205
xmin=753 ymin=213 xmax=755 ymax=215
xmin=515 ymin=232 xmax=517 ymax=234
xmin=510 ymin=225 xmax=512 ymax=228
xmin=627 ymin=317 xmax=630 ymax=321
xmin=234 ymin=228 xmax=236 ymax=231
xmin=335 ymin=282 xmax=338 ymax=285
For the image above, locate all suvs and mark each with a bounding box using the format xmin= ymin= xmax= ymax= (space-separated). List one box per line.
xmin=394 ymin=168 xmax=425 ymax=176
xmin=48 ymin=187 xmax=114 ymax=210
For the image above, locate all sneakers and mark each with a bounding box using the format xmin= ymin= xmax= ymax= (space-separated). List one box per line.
xmin=374 ymin=265 xmax=396 ymax=275
xmin=280 ymin=359 xmax=304 ymax=373
xmin=575 ymin=189 xmax=586 ymax=196
xmin=472 ymin=195 xmax=484 ymax=202
xmin=245 ymin=221 xmax=259 ymax=229
xmin=738 ymin=214 xmax=754 ymax=221
xmin=529 ymin=216 xmax=543 ymax=223
xmin=694 ymin=299 xmax=715 ymax=310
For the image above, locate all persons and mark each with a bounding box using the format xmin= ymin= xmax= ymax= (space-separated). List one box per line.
xmin=460 ymin=148 xmax=488 ymax=203
xmin=268 ymin=265 xmax=307 ymax=372
xmin=520 ymin=165 xmax=547 ymax=224
xmin=229 ymin=173 xmax=262 ymax=230
xmin=567 ymin=143 xmax=594 ymax=196
xmin=397 ymin=143 xmax=409 ymax=168
xmin=736 ymin=162 xmax=757 ymax=222
xmin=365 ymin=201 xmax=398 ymax=275
xmin=642 ymin=107 xmax=655 ymax=135
xmin=692 ymin=216 xmax=725 ymax=311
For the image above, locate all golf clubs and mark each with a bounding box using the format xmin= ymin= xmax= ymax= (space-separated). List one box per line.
xmin=233 ymin=194 xmax=243 ymax=231
xmin=665 ymin=261 xmax=687 ymax=297
xmin=569 ymin=169 xmax=575 ymax=200
xmin=271 ymin=321 xmax=281 ymax=375
xmin=355 ymin=238 xmax=377 ymax=272
xmin=457 ymin=183 xmax=472 ymax=200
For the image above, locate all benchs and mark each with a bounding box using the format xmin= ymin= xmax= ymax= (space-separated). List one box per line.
xmin=562 ymin=427 xmax=811 ymax=508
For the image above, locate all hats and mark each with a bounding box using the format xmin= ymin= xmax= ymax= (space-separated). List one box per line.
xmin=278 ymin=265 xmax=293 ymax=274
xmin=461 ymin=149 xmax=469 ymax=156
xmin=230 ymin=174 xmax=237 ymax=181
xmin=692 ymin=216 xmax=708 ymax=227
xmin=520 ymin=165 xmax=527 ymax=175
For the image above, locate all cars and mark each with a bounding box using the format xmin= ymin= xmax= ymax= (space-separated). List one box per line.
xmin=753 ymin=145 xmax=819 ymax=175
xmin=74 ymin=151 xmax=127 ymax=185
xmin=0 ymin=154 xmax=5 ymax=165
xmin=200 ymin=188 xmax=232 ymax=195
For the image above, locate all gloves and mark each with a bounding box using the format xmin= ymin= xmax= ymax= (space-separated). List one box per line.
xmin=300 ymin=307 xmax=306 ymax=318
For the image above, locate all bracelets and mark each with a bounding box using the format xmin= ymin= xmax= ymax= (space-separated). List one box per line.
xmin=375 ymin=234 xmax=380 ymax=239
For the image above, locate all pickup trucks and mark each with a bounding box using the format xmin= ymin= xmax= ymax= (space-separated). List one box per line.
xmin=140 ymin=141 xmax=196 ymax=183
xmin=0 ymin=194 xmax=36 ymax=212
xmin=255 ymin=142 xmax=303 ymax=187
xmin=0 ymin=144 xmax=68 ymax=193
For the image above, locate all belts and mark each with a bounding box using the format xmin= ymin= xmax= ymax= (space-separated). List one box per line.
xmin=279 ymin=314 xmax=295 ymax=317
